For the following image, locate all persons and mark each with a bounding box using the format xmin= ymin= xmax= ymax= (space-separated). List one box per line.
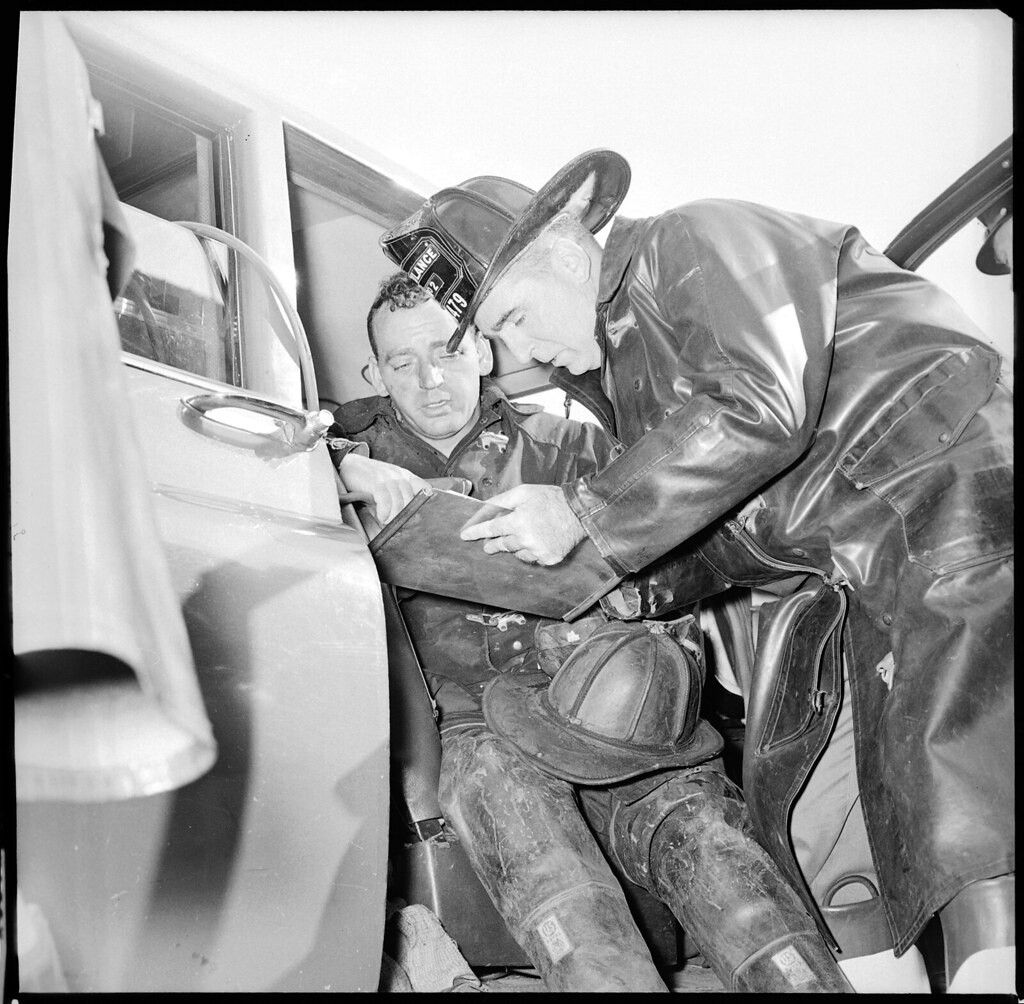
xmin=321 ymin=268 xmax=854 ymax=994
xmin=380 ymin=147 xmax=1015 ymax=993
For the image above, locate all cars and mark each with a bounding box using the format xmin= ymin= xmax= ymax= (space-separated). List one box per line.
xmin=10 ymin=10 xmax=1013 ymax=994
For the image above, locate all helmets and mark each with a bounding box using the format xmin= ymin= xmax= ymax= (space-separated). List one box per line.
xmin=480 ymin=621 xmax=725 ymax=789
xmin=381 ymin=145 xmax=636 ymax=357
